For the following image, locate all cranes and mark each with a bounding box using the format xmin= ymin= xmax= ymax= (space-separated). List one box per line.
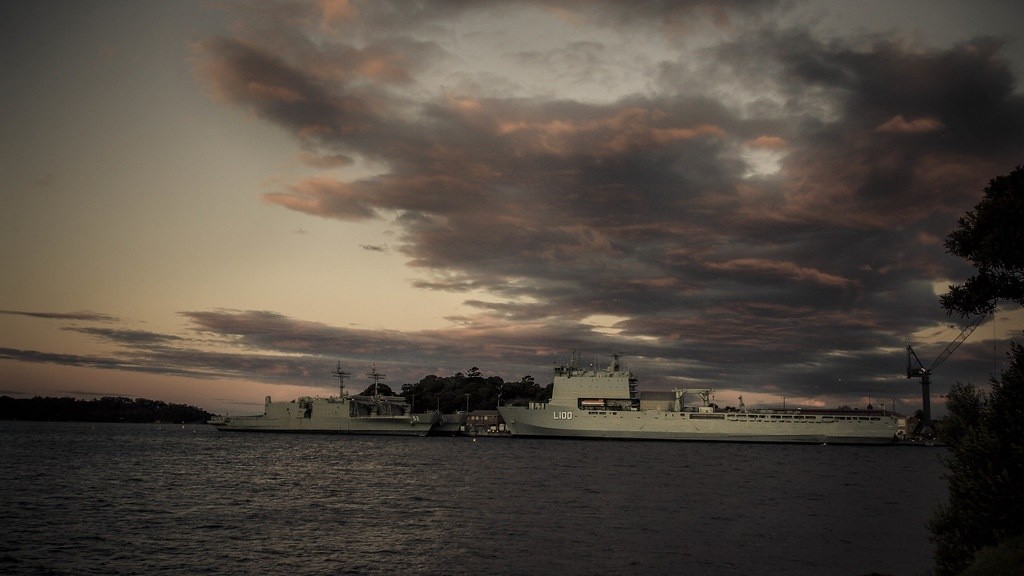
xmin=906 ymin=312 xmax=997 ymax=425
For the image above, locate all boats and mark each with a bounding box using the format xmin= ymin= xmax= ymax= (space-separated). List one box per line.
xmin=206 ymin=361 xmax=439 ymax=437
xmin=497 ymin=348 xmax=898 ymax=446
xmin=367 ymin=362 xmax=470 ymax=438
